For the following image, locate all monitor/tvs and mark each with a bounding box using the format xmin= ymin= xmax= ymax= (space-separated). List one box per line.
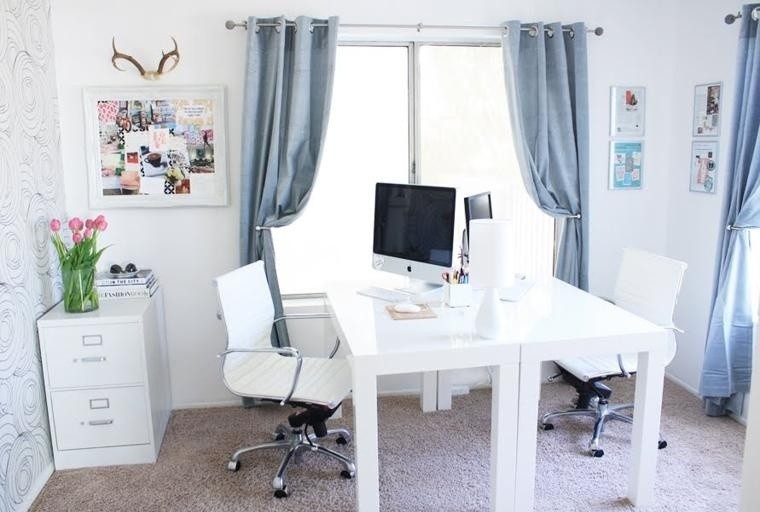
xmin=464 ymin=191 xmax=493 ymax=251
xmin=372 ymin=182 xmax=462 ymax=295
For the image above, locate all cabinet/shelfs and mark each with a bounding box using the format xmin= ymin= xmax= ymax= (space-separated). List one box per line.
xmin=36 ymin=287 xmax=172 ymax=471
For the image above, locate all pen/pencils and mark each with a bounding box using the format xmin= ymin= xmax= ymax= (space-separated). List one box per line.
xmin=442 ymin=267 xmax=469 ymax=284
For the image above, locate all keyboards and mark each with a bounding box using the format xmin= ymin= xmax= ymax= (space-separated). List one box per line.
xmin=500 ymin=278 xmax=538 ymax=302
xmin=357 ymin=286 xmax=408 ymax=302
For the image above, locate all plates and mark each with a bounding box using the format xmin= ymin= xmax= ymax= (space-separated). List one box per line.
xmin=108 ymin=271 xmax=143 ymax=277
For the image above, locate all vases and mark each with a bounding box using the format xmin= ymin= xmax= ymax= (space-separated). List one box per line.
xmin=62 ymin=268 xmax=99 ymax=312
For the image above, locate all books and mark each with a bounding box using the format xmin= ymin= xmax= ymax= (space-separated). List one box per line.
xmin=94 ymin=268 xmax=160 ymax=298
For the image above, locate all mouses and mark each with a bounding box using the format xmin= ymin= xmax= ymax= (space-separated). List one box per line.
xmin=394 ymin=303 xmax=421 ymax=312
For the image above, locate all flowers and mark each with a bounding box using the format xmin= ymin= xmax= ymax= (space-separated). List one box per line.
xmin=48 ymin=214 xmax=116 ymax=312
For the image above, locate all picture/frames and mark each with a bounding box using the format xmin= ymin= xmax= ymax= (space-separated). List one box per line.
xmin=609 ymin=85 xmax=647 ymax=190
xmin=81 ymin=84 xmax=229 ymax=208
xmin=689 ymin=81 xmax=724 ymax=194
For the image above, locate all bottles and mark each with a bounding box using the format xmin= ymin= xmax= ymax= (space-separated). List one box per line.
xmin=475 ymin=287 xmax=502 ymax=341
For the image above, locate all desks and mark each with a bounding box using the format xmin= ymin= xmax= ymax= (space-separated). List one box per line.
xmin=325 ymin=259 xmax=677 ymax=512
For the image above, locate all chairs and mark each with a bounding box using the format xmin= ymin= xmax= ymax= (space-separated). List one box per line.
xmin=539 ymin=246 xmax=688 ymax=457
xmin=213 ymin=259 xmax=355 ymax=497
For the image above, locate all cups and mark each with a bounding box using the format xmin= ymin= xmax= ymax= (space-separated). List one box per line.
xmin=144 ymin=154 xmax=163 ymax=167
